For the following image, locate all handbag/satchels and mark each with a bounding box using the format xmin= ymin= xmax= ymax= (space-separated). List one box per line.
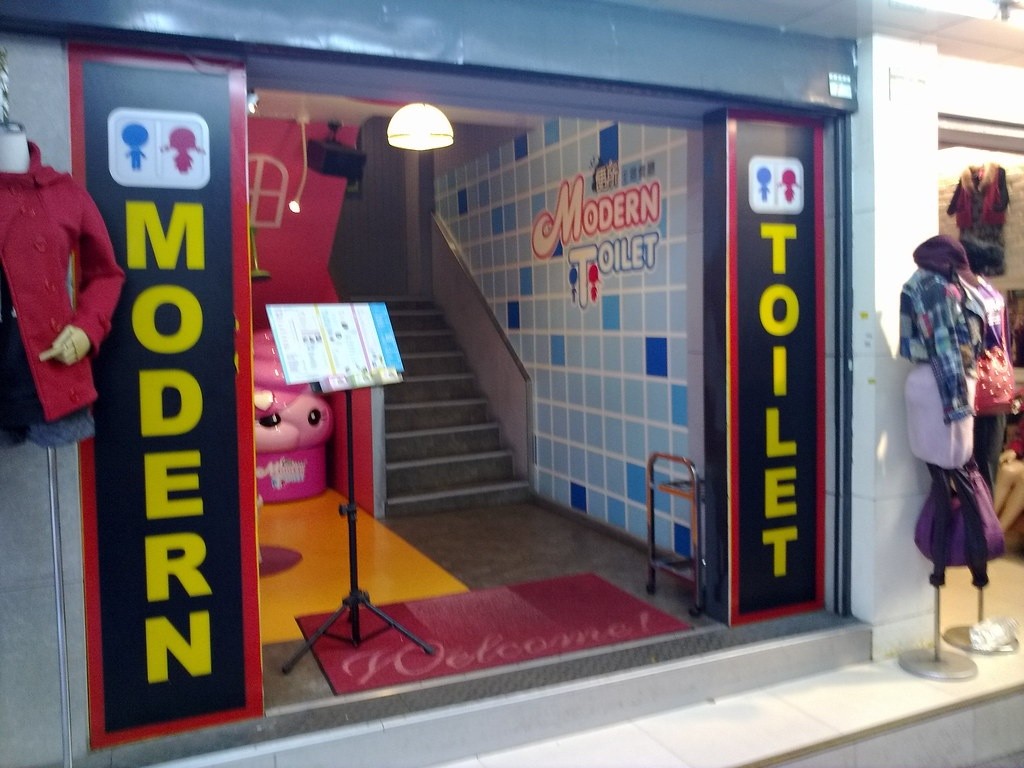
xmin=976 ymin=346 xmax=1016 ymax=417
xmin=914 ymin=472 xmax=1007 ymax=567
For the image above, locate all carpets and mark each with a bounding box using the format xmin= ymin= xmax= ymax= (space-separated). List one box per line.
xmin=294 ymin=569 xmax=694 ymax=697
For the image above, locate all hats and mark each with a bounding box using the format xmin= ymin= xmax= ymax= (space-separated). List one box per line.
xmin=912 ymin=234 xmax=973 ymax=280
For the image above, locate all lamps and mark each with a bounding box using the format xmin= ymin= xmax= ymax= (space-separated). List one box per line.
xmin=247 ymin=92 xmax=260 ymax=114
xmin=289 ymin=114 xmax=310 ymax=214
xmin=387 ymin=102 xmax=454 ymax=151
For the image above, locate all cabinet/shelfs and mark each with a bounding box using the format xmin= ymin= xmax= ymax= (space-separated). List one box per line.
xmin=645 ymin=451 xmax=702 ymax=618
